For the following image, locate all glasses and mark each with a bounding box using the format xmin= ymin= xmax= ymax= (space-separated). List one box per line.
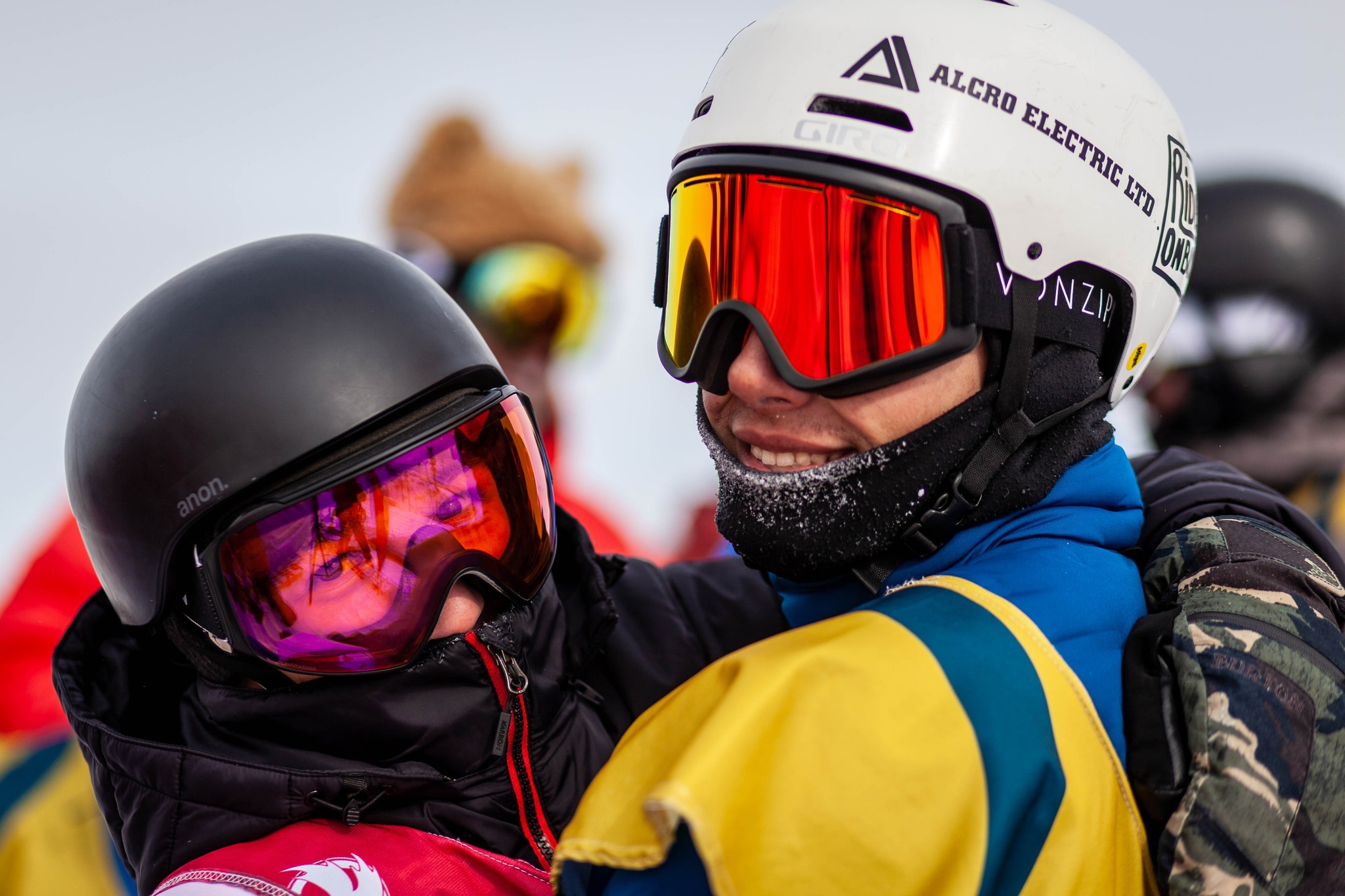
xmin=204 ymin=380 xmax=558 ymax=680
xmin=658 ymin=156 xmax=983 ymax=400
xmin=391 ymin=237 xmax=592 ymax=353
xmin=1141 ymin=297 xmax=1309 ymax=393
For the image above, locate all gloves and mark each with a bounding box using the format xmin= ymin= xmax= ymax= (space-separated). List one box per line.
xmin=1139 ymin=516 xmax=1345 ymax=896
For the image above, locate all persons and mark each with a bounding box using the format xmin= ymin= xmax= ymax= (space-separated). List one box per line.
xmin=1137 ymin=180 xmax=1345 ymax=545
xmin=552 ymin=0 xmax=1199 ymax=896
xmin=53 ymin=236 xmax=1345 ymax=896
xmin=0 ymin=118 xmax=600 ymax=895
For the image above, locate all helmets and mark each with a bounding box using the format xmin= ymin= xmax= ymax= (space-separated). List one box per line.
xmin=63 ymin=232 xmax=512 ymax=622
xmin=670 ymin=0 xmax=1201 ymax=404
xmin=1138 ymin=183 xmax=1344 ymax=414
